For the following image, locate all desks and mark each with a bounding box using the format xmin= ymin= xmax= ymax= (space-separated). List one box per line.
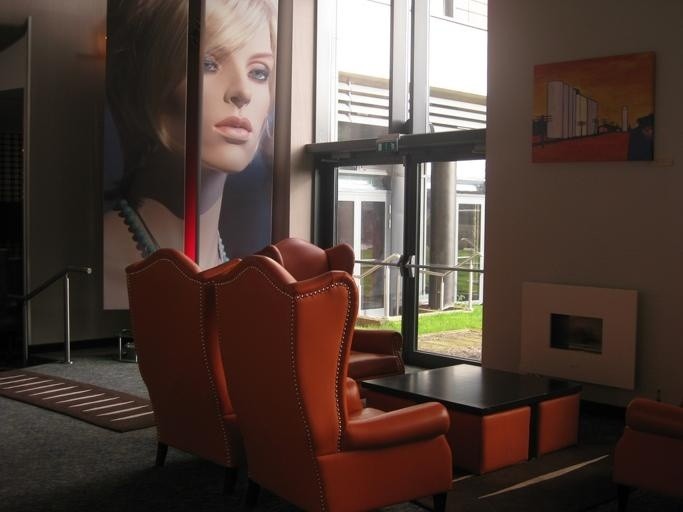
xmin=360 ymin=362 xmax=582 ymax=473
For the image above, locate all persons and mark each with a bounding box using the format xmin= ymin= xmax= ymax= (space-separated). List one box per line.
xmin=101 ymin=1 xmax=277 ymax=310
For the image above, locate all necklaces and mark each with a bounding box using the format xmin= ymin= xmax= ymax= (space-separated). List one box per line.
xmin=112 ymin=198 xmax=229 ymax=274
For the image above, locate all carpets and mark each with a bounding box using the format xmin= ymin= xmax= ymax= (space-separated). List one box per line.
xmin=409 ymin=398 xmax=682 ymax=510
xmin=0 ymin=367 xmax=157 ymax=432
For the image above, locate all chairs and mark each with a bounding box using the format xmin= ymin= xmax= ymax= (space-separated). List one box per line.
xmin=608 ymin=396 xmax=682 ymax=511
xmin=124 ymin=237 xmax=454 ymax=510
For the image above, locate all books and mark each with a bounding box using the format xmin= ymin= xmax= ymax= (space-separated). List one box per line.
xmin=554 ymin=338 xmax=601 ymax=355
